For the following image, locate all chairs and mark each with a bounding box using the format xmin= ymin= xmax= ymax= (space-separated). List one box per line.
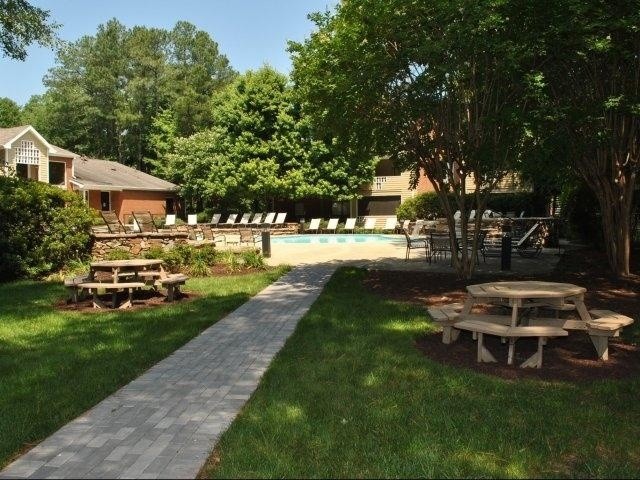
xmin=404 ymin=229 xmax=487 ymax=266
xmin=100 ymin=209 xmax=286 ymax=245
xmin=305 ymin=217 xmax=397 ymax=234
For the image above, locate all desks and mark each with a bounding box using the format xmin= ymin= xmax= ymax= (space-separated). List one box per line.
xmin=452 ymin=280 xmax=590 ymax=366
xmin=89 ymin=259 xmax=166 ymax=308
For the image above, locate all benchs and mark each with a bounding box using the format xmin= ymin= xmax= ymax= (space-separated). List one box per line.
xmin=62 ymin=271 xmax=190 ymax=310
xmin=428 ymin=302 xmax=636 ymax=369
